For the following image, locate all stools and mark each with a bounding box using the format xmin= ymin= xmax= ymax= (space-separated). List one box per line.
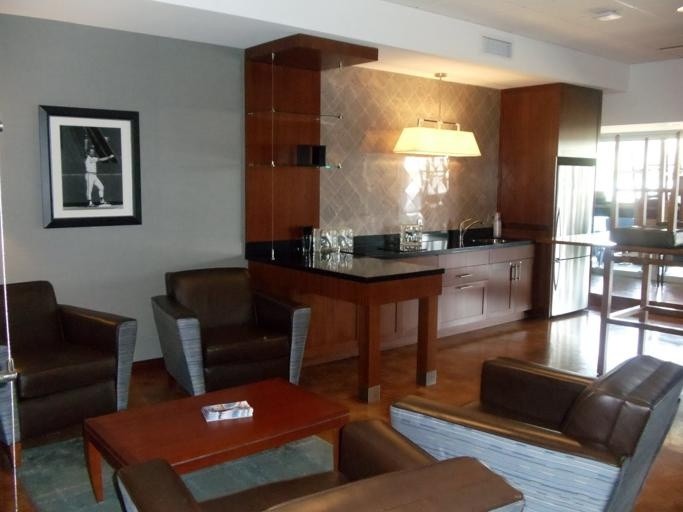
xmin=610 ymin=132 xmax=682 ymax=247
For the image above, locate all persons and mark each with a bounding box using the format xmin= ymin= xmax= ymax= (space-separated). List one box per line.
xmin=82 ymin=133 xmax=115 ymax=208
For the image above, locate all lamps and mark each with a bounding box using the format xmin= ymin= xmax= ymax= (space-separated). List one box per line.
xmin=391 ymin=68 xmax=481 ymax=160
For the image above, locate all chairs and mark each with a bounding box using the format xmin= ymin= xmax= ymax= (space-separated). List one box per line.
xmin=387 ymin=355 xmax=681 ymax=512
xmin=118 ymin=408 xmax=524 ymax=512
xmin=0 ymin=278 xmax=137 ymax=468
xmin=149 ymin=263 xmax=312 ymax=390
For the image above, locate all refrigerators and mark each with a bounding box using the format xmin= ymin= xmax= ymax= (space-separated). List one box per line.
xmin=550 ymin=165 xmax=596 ymax=316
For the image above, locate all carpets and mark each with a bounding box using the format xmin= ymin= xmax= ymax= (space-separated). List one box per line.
xmin=3 ymin=423 xmax=334 ymax=509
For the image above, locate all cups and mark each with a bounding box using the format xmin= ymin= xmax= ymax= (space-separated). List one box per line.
xmin=303 ymin=253 xmax=352 ymax=271
xmin=295 ymin=143 xmax=327 ymax=166
xmin=399 ymin=224 xmax=423 ymax=246
xmin=300 ymin=225 xmax=354 ymax=253
xmin=399 ymin=245 xmax=423 ymax=255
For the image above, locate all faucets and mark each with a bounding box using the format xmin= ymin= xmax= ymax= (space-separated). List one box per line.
xmin=457 ymin=217 xmax=485 ymax=241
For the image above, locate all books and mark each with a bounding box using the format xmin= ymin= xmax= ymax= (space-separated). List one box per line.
xmin=201 ymin=400 xmax=254 ymax=424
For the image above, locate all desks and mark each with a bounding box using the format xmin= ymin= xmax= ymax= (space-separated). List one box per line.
xmin=281 ymin=255 xmax=444 ymax=403
xmin=534 ymin=233 xmax=683 ymax=378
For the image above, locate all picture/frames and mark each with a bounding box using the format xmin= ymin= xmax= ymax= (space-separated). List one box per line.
xmin=38 ymin=103 xmax=142 ymax=229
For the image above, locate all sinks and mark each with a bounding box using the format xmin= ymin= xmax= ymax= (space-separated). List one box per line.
xmin=472 ymin=238 xmax=508 ymax=245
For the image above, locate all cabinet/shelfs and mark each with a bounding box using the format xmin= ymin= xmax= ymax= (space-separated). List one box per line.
xmin=483 ymin=242 xmax=532 ymax=336
xmin=378 ymin=254 xmax=436 ymax=342
xmin=434 ymin=246 xmax=485 ymax=338
xmin=240 ymin=32 xmax=378 ymax=261
xmin=290 ymin=269 xmax=359 ymax=365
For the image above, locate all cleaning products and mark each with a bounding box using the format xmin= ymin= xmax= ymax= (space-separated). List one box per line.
xmin=492 ymin=212 xmax=503 ymax=239
